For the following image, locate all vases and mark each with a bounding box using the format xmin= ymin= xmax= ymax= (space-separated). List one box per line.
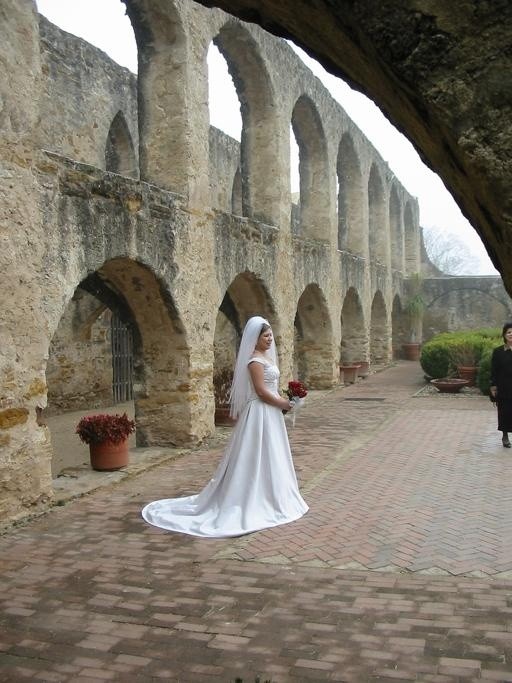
xmin=89 ymin=434 xmax=130 ymax=470
xmin=340 ymin=365 xmax=362 ymax=384
xmin=457 ymin=366 xmax=475 ymax=386
xmin=344 ymin=360 xmax=369 ymax=376
xmin=215 ymin=408 xmax=239 ymax=426
xmin=430 ymin=378 xmax=470 ymax=392
xmin=401 ymin=343 xmax=420 ymax=361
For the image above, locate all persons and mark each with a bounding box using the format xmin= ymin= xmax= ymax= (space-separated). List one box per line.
xmin=489 ymin=322 xmax=511 ymax=448
xmin=141 ymin=315 xmax=310 ymax=538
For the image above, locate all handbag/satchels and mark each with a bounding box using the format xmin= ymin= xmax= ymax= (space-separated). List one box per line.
xmin=490 ymin=383 xmax=503 ymax=402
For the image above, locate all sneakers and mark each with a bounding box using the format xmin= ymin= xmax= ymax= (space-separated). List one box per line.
xmin=502 ymin=438 xmax=510 ymax=447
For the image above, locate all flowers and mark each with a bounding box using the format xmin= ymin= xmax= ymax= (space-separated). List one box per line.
xmin=282 ymin=382 xmax=308 ymax=414
xmin=214 ymin=367 xmax=234 ymax=407
xmin=75 ymin=410 xmax=139 ymax=447
